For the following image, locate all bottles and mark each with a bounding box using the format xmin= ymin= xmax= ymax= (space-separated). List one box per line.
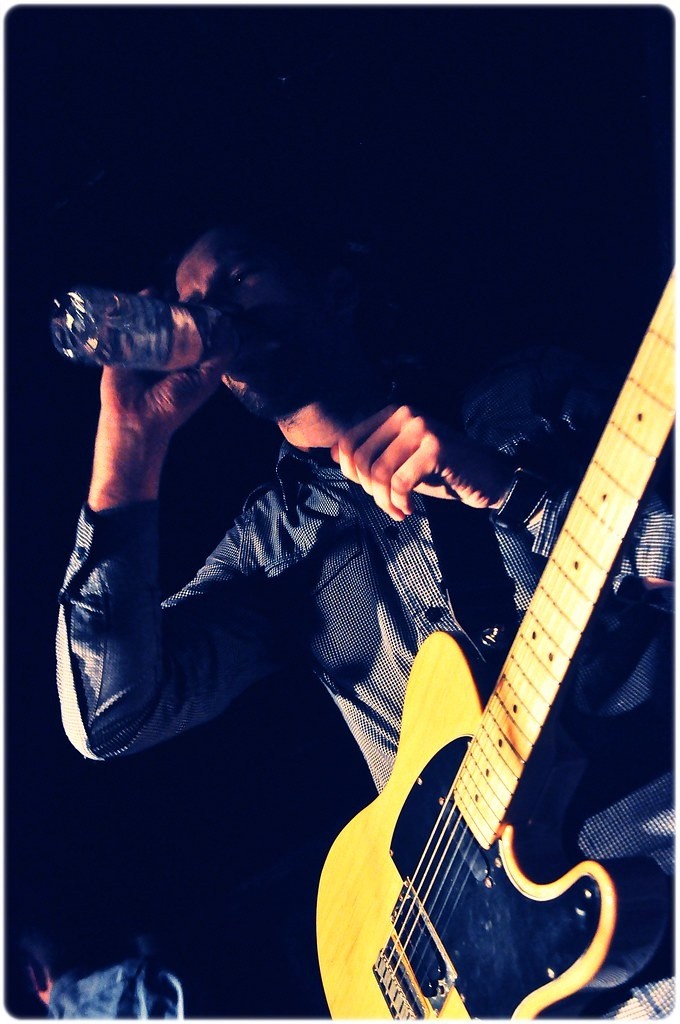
xmin=51 ymin=285 xmax=227 ymax=372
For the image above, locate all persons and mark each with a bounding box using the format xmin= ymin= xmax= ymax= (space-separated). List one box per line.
xmin=52 ymin=192 xmax=672 ymax=1019
xmin=23 ymin=909 xmax=184 ymax=1020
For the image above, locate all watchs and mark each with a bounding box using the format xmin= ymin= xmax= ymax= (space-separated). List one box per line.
xmin=491 ymin=455 xmax=549 ymax=536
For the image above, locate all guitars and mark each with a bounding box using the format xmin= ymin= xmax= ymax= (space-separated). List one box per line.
xmin=315 ymin=266 xmax=674 ymax=1021
